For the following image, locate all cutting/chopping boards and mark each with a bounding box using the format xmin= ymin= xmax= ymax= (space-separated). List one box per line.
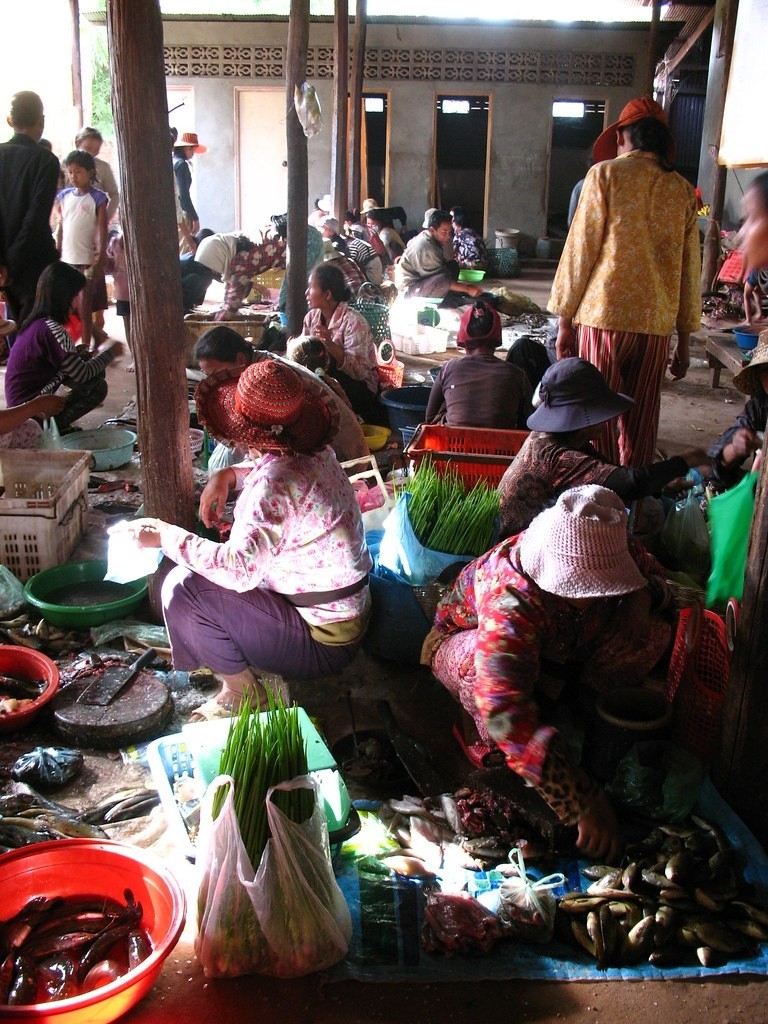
xmin=49 ymin=667 xmax=174 ymax=750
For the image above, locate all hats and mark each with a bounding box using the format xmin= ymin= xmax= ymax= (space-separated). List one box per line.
xmin=593 ymin=97 xmax=668 ymax=164
xmin=174 ymin=132 xmax=207 ymax=154
xmin=422 ymin=208 xmax=439 ymax=228
xmin=317 ymin=194 xmax=335 ymax=211
xmin=192 ymin=361 xmax=341 ymax=457
xmin=520 ymin=483 xmax=649 ymax=599
xmin=526 ymin=356 xmax=635 ymax=433
xmin=360 ymin=198 xmax=385 ymax=214
xmin=732 ymin=330 xmax=768 ymax=396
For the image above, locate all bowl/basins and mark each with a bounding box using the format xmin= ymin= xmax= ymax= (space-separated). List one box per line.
xmin=361 ymin=423 xmax=392 ymax=451
xmin=60 ymin=429 xmax=137 ymax=471
xmin=732 ymin=326 xmax=758 ymax=349
xmin=0 ymin=644 xmax=59 ymax=725
xmin=22 ymin=560 xmax=148 ymax=632
xmin=458 ymin=270 xmax=486 ymax=282
xmin=0 ymin=837 xmax=188 ymax=1024
xmin=378 ymin=385 xmax=432 ymax=434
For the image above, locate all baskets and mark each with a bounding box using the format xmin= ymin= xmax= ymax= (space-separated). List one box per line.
xmin=388 ymin=322 xmax=449 ymax=356
xmin=189 ymin=428 xmax=203 ymax=452
xmin=667 ymin=597 xmax=740 ymax=720
xmin=246 ymin=267 xmax=287 ymax=304
xmin=346 ymin=281 xmax=391 ymax=341
xmin=486 ymin=235 xmax=520 ymax=278
xmin=372 ymin=341 xmax=404 ymax=389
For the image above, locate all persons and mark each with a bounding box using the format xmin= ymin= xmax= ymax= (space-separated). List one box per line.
xmin=420 ymin=484 xmax=667 ymax=864
xmin=323 ymin=238 xmax=372 ymax=300
xmin=286 ymin=336 xmax=350 ymax=410
xmin=5 ymin=261 xmax=125 ymax=436
xmin=309 ymin=194 xmax=407 ymax=286
xmin=193 ymin=325 xmax=369 ymax=475
xmin=0 ymin=90 xmax=65 ymax=365
xmin=300 ymin=264 xmax=379 ymax=412
xmin=55 ymin=149 xmax=107 ymax=352
xmin=496 ymin=358 xmax=708 ymax=540
xmin=57 ymin=127 xmax=119 ymax=356
xmin=546 ymin=94 xmax=702 ymax=468
xmin=394 ymin=206 xmax=486 ymax=298
xmin=507 ymin=320 xmax=559 ymax=390
xmin=126 ymin=361 xmax=366 ymax=724
xmin=425 ymin=301 xmax=534 ymax=432
xmin=174 ymin=133 xmax=324 ymax=322
xmin=707 ymin=172 xmax=768 ymax=478
xmin=104 ymin=234 xmax=136 ymax=374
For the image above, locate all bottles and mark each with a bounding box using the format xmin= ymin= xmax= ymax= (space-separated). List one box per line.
xmin=536 ymin=237 xmax=552 ymax=258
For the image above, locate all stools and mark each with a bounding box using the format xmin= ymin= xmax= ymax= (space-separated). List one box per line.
xmin=275 ymin=673 xmax=362 ymax=761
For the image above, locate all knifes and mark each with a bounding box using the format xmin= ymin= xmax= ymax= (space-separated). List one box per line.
xmin=74 ymin=648 xmax=157 ymax=706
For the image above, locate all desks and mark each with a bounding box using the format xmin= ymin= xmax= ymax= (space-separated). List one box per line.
xmin=705 ymin=334 xmax=752 ymax=388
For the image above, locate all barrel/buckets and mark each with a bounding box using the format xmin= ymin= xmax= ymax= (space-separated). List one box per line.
xmin=494 ymin=228 xmax=520 ymax=251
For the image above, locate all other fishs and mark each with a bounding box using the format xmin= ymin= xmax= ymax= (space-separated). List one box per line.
xmin=387 ymin=794 xmax=768 ymax=972
xmin=0 ymin=891 xmax=152 ymax=1008
xmin=0 ymin=778 xmax=159 ymax=853
xmin=0 ymin=604 xmax=213 ymax=715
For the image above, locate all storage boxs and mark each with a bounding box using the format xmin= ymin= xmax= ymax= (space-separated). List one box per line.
xmin=399 ymin=422 xmax=531 ymax=499
xmin=0 ymin=446 xmax=94 ymax=585
xmin=184 ymin=312 xmax=272 ymax=367
xmin=390 ymin=324 xmax=449 ymax=355
xmin=247 ymin=268 xmax=286 ymax=303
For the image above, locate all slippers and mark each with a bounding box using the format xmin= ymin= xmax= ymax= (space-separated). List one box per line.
xmin=188 ymin=694 xmax=279 ymax=726
xmin=451 ymin=717 xmax=505 ymax=771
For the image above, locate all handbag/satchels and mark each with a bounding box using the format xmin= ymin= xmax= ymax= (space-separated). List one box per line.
xmin=192 ymin=774 xmax=354 ymax=980
